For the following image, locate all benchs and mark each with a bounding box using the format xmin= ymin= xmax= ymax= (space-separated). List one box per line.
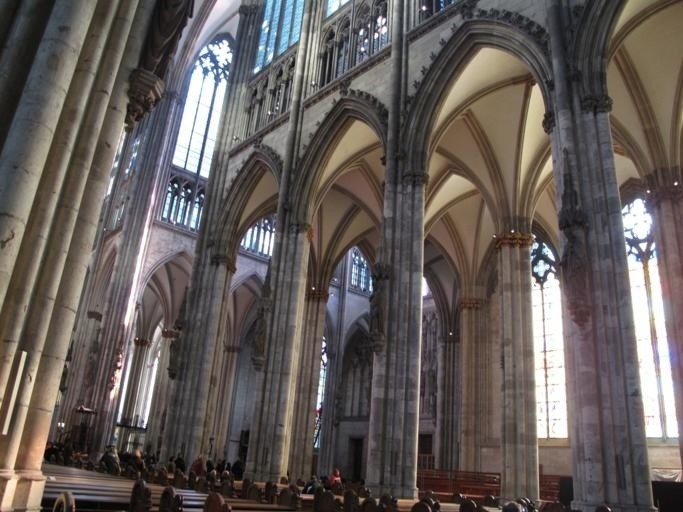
xmin=39 ymin=461 xmax=574 ymax=512
